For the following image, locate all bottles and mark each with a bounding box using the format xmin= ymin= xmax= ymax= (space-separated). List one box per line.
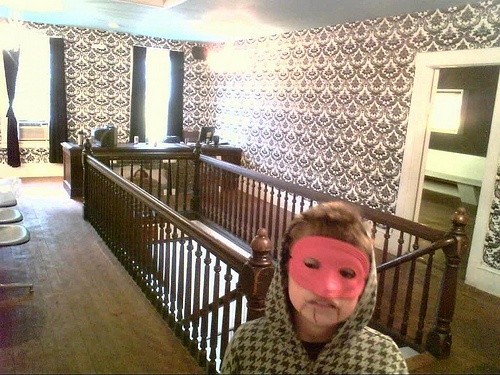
xmin=77 ymin=131 xmax=84 ymax=146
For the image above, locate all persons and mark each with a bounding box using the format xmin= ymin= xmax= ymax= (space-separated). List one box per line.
xmin=217 ymin=201 xmax=411 ymax=375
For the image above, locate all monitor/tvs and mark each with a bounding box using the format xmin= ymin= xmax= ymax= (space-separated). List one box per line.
xmin=199 ymin=126 xmax=215 ymax=147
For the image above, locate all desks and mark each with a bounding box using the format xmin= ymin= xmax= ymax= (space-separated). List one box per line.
xmin=61 ymin=139 xmax=245 ymax=200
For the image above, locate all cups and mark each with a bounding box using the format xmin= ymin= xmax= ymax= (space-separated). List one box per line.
xmin=134 ymin=136 xmax=138 ymax=143
xmin=185 ymin=138 xmax=189 ymax=143
